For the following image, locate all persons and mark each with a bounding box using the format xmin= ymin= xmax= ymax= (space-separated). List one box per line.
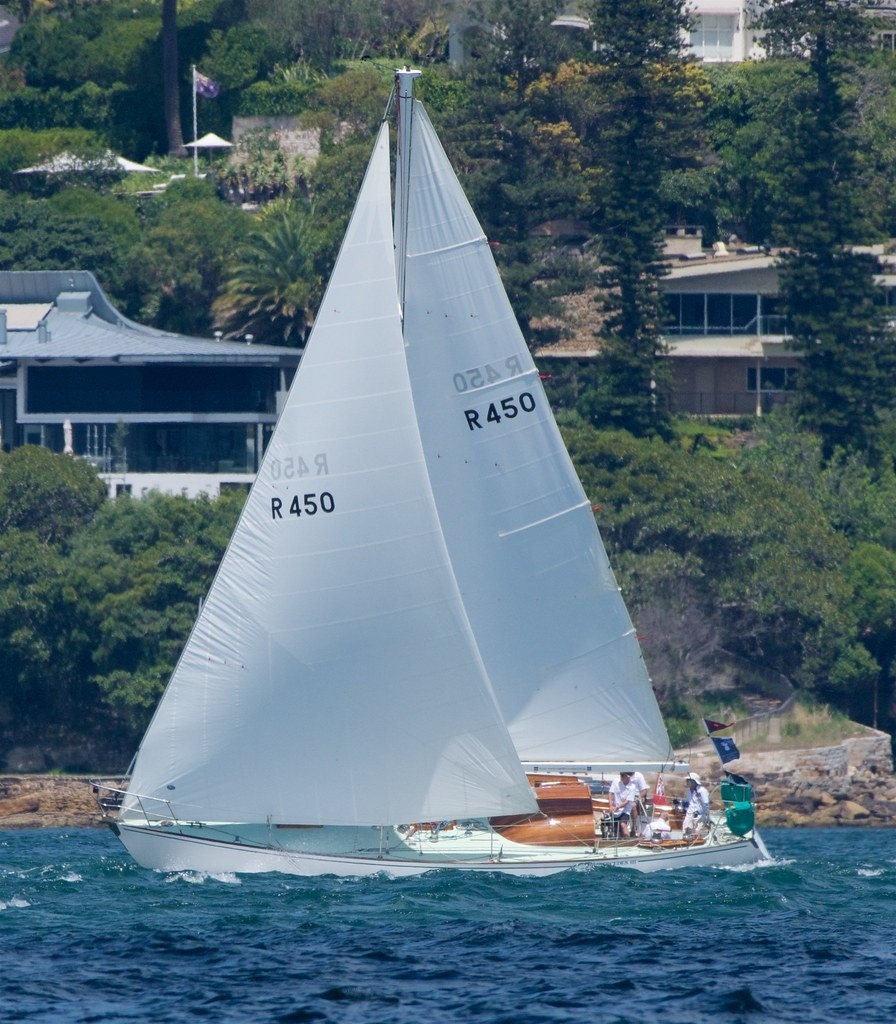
xmin=609 ymin=771 xmax=650 ymax=837
xmin=682 ymin=772 xmax=710 ymax=834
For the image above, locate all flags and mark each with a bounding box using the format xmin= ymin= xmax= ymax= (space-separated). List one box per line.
xmin=194 ymin=70 xmax=220 ymax=99
xmin=705 ymin=717 xmax=741 ymax=767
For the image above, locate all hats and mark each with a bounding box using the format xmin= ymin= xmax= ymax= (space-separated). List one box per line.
xmin=684 ymin=773 xmax=701 ymax=785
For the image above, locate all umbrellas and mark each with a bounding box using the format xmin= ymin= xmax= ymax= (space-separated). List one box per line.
xmin=179 ymin=132 xmax=234 ymax=174
xmin=14 ymin=149 xmax=164 ymax=173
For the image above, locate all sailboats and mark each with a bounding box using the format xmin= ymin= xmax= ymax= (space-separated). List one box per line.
xmin=86 ymin=72 xmax=792 ymax=873
